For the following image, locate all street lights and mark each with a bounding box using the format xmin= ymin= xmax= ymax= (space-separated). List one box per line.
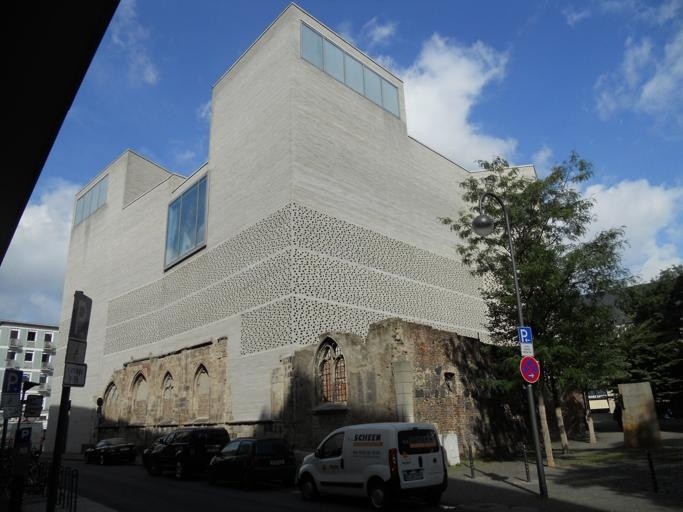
xmin=471 ymin=190 xmax=549 ymax=511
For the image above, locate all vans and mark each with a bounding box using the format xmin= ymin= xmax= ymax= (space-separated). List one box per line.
xmin=294 ymin=422 xmax=448 ymax=509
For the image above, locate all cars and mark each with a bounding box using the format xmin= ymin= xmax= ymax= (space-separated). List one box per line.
xmin=82 ymin=437 xmax=137 ymax=465
xmin=208 ymin=437 xmax=295 ymax=489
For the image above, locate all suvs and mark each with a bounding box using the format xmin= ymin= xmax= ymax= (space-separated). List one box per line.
xmin=141 ymin=428 xmax=230 ymax=479
